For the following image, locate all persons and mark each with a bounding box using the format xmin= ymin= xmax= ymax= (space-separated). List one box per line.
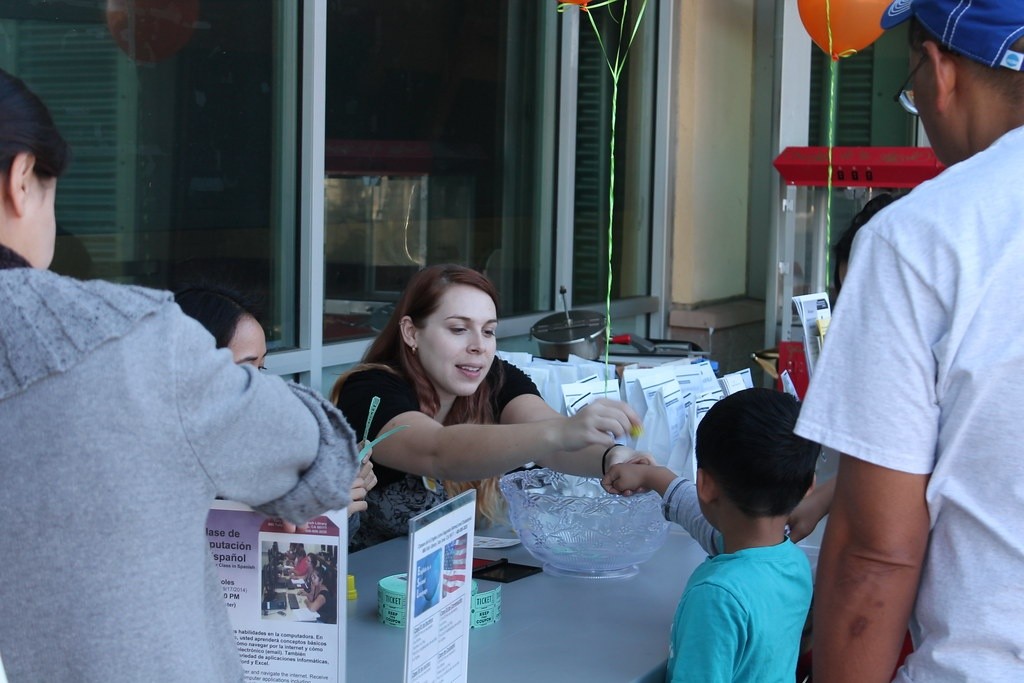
xmin=600 ymin=388 xmax=822 ymax=683
xmin=324 ymin=264 xmax=657 ymax=554
xmin=272 ymin=541 xmax=336 ymax=624
xmin=176 ymin=282 xmax=377 ymax=521
xmin=794 ymin=0 xmax=1024 ymax=683
xmin=0 ymin=71 xmax=358 ymax=683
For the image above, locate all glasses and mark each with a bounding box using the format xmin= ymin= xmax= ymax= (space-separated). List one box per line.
xmin=894 ymin=44 xmax=943 ymax=116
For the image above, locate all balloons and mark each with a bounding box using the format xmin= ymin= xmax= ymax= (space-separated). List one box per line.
xmin=798 ymin=0 xmax=893 ymax=62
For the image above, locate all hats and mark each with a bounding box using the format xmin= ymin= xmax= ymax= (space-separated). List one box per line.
xmin=882 ymin=0 xmax=1024 ymax=73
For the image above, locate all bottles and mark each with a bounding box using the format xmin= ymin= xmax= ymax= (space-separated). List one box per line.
xmin=691 ymin=358 xmax=721 ymax=379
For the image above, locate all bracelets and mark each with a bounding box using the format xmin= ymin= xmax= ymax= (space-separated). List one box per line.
xmin=602 ymin=443 xmax=623 ymax=476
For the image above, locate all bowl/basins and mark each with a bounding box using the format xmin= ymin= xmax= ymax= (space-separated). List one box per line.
xmin=498 ymin=468 xmax=669 ymax=581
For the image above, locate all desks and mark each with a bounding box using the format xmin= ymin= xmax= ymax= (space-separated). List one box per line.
xmin=341 ymin=444 xmax=845 ymax=682
